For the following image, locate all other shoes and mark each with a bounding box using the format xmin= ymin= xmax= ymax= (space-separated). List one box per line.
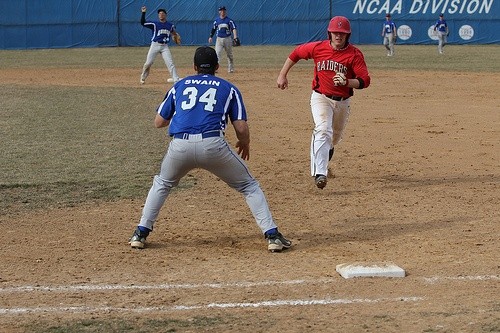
xmin=315 ymin=174 xmax=327 ymax=189
xmin=387 ymin=51 xmax=390 ymax=56
xmin=267 ymin=232 xmax=292 ymax=251
xmin=440 ymin=51 xmax=443 ymax=53
xmin=141 ymin=78 xmax=145 ymax=84
xmin=128 ymin=230 xmax=146 ymax=248
xmin=174 ymin=81 xmax=179 ymax=84
xmin=228 ymin=68 xmax=233 ymax=73
xmin=329 ymin=147 xmax=334 ymax=161
xmin=390 ymin=51 xmax=393 ymax=56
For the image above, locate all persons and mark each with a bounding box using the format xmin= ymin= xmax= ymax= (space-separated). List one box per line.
xmin=208 ymin=6 xmax=240 ymax=73
xmin=140 ymin=6 xmax=179 ymax=84
xmin=276 ymin=16 xmax=371 ymax=189
xmin=434 ymin=14 xmax=448 ymax=54
xmin=381 ymin=14 xmax=396 ymax=56
xmin=130 ymin=47 xmax=292 ymax=250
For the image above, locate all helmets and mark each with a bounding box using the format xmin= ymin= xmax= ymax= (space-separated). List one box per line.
xmin=327 ymin=16 xmax=351 ymax=40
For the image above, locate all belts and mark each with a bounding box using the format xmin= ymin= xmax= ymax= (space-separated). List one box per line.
xmin=174 ymin=131 xmax=220 ymax=139
xmin=154 ymin=41 xmax=167 ymax=44
xmin=315 ymin=89 xmax=349 ymax=101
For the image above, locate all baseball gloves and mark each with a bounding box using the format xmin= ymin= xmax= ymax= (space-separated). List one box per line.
xmin=232 ymin=38 xmax=240 ymax=46
xmin=173 ymin=33 xmax=181 ymax=44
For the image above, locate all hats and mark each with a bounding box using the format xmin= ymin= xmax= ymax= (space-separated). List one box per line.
xmin=219 ymin=6 xmax=226 ymax=11
xmin=439 ymin=14 xmax=443 ymax=17
xmin=385 ymin=14 xmax=391 ymax=17
xmin=194 ymin=46 xmax=218 ymax=69
xmin=157 ymin=8 xmax=166 ymax=14
